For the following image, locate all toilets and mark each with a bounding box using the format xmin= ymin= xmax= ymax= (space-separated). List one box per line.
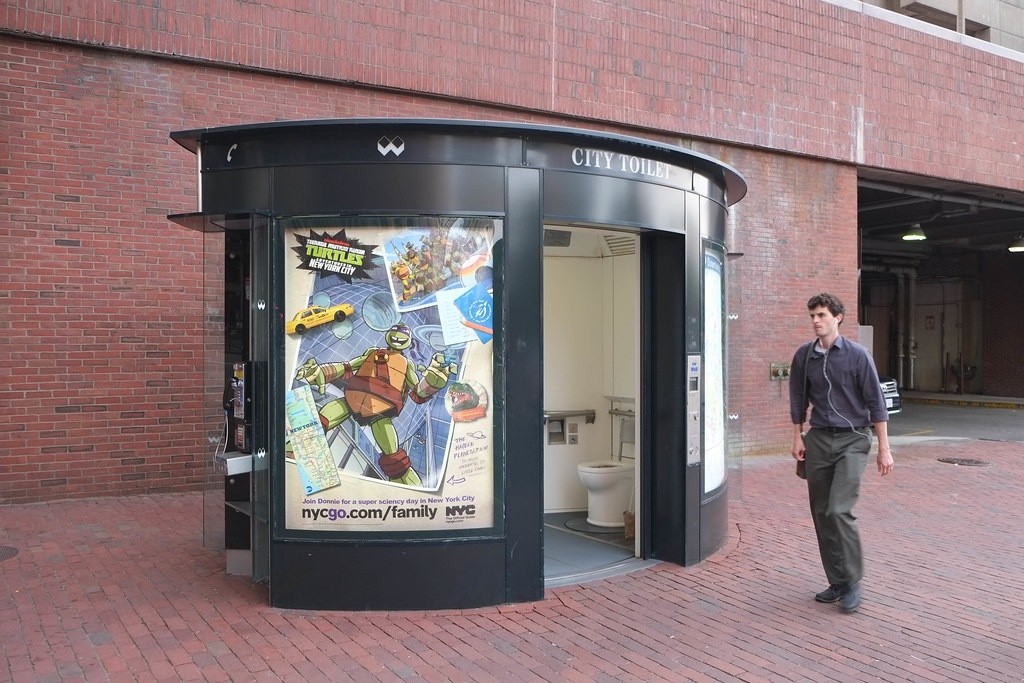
xmin=577 ymin=460 xmax=636 ymax=528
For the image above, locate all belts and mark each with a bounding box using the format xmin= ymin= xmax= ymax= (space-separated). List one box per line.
xmin=822 ymin=426 xmax=864 ymax=432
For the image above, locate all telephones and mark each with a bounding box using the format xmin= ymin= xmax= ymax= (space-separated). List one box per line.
xmin=222 ymin=360 xmax=266 ymax=418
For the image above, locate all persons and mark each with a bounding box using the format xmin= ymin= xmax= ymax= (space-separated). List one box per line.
xmin=789 ymin=292 xmax=895 ymax=612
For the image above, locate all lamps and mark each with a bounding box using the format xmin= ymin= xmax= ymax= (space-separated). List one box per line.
xmin=1007 ymin=233 xmax=1024 ymax=254
xmin=895 ymin=222 xmax=933 ymax=246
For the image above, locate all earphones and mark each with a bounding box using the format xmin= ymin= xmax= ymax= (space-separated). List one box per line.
xmin=835 ymin=319 xmax=839 ymax=325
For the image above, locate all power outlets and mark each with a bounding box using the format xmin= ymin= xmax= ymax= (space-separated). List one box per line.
xmin=568 ymin=423 xmax=578 ymax=445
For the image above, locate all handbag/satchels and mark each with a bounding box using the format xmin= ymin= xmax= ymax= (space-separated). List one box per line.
xmin=797 ymin=432 xmax=807 ymax=478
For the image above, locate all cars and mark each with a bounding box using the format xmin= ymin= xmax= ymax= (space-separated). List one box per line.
xmin=285 ymin=302 xmax=354 ymax=335
xmin=879 ymin=375 xmax=903 ymax=415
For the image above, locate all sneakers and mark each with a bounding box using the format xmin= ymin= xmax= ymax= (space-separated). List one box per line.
xmin=841 ymin=583 xmax=860 ymax=612
xmin=815 ymin=583 xmax=846 ymax=602
